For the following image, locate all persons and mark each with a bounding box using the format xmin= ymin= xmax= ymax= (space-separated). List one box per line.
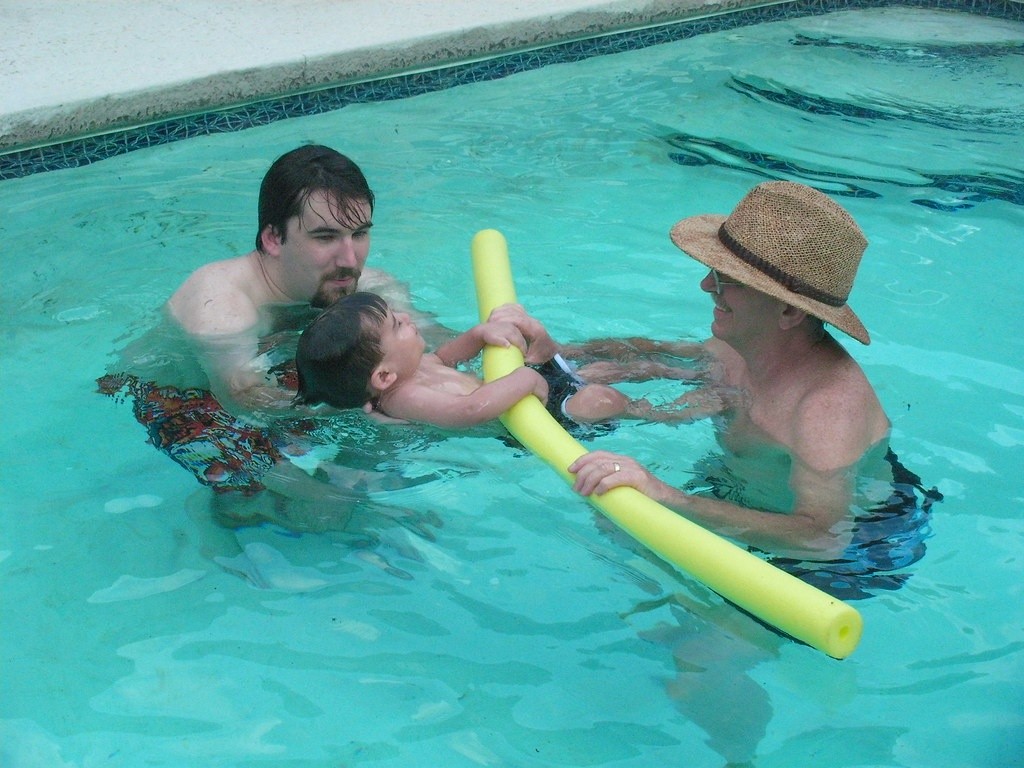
xmin=295 ymin=292 xmax=625 ymax=428
xmin=169 ymin=144 xmax=374 ymax=339
xmin=489 ymin=180 xmax=888 ymax=499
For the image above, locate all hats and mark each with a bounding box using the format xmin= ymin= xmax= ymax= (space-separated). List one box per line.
xmin=670 ymin=181 xmax=871 ymax=345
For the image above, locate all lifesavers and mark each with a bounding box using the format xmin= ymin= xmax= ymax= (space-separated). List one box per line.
xmin=468 ymin=227 xmax=864 ymax=661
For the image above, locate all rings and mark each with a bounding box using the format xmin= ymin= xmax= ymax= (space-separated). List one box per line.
xmin=614 ymin=462 xmax=620 ymax=472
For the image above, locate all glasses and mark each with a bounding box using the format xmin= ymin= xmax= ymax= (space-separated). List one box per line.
xmin=712 ymin=269 xmax=745 ymax=295
